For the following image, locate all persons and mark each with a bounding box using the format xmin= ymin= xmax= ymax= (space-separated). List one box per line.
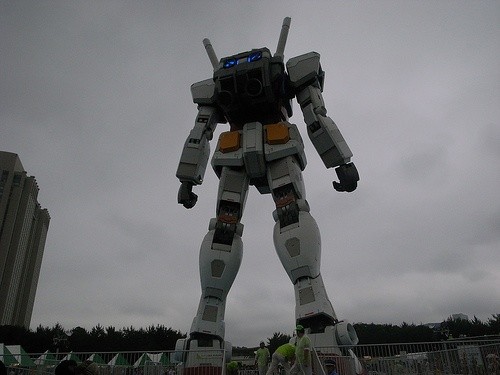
xmin=177 ymin=42 xmax=366 ymax=373
xmin=255 ymin=340 xmax=271 ymax=375
xmin=56 ymin=359 xmax=100 ymax=375
xmin=293 ymin=325 xmax=313 ymax=375
xmin=324 ymin=359 xmax=338 ymax=375
xmin=226 ymin=360 xmax=244 ymax=375
xmin=265 ymin=342 xmax=297 ymax=375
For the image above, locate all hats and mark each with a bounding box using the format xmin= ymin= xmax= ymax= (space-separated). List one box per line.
xmin=294 ymin=325 xmax=303 ymax=330
xmin=324 ymin=359 xmax=336 ymax=367
